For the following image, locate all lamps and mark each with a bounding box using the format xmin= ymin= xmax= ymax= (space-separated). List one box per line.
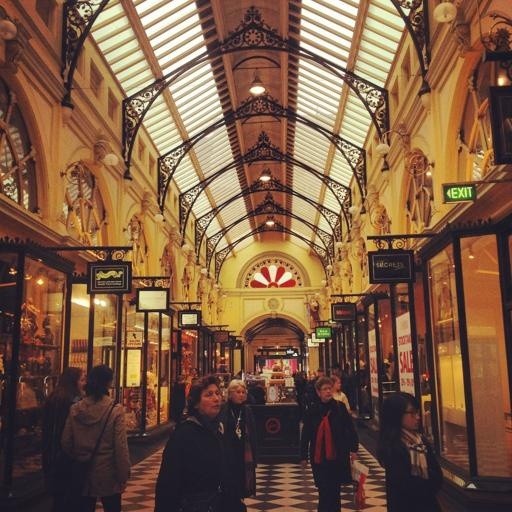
xmin=248 ymin=68 xmax=280 ymax=226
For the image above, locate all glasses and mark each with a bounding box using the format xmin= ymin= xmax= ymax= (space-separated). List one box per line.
xmin=406 ymin=408 xmax=421 ymax=418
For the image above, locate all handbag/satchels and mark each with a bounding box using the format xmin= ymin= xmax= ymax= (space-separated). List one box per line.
xmin=348 ymin=452 xmax=371 ymax=511
xmin=63 ymin=454 xmax=95 ymax=512
xmin=179 ymin=490 xmax=223 ymax=512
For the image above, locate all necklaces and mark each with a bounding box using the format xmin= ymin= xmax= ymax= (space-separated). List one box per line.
xmin=230 ymin=407 xmax=242 ymax=439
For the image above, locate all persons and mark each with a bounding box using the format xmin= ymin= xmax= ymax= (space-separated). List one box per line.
xmin=216 ymin=376 xmax=258 ymax=512
xmin=152 ymin=371 xmax=251 ymax=512
xmin=378 ymin=391 xmax=448 ymax=511
xmin=172 ymin=372 xmax=187 ymax=419
xmin=297 ymin=376 xmax=361 ymax=511
xmin=294 ymin=365 xmax=360 ymax=426
xmin=38 ymin=366 xmax=84 ymax=511
xmin=57 ymin=362 xmax=133 ymax=511
